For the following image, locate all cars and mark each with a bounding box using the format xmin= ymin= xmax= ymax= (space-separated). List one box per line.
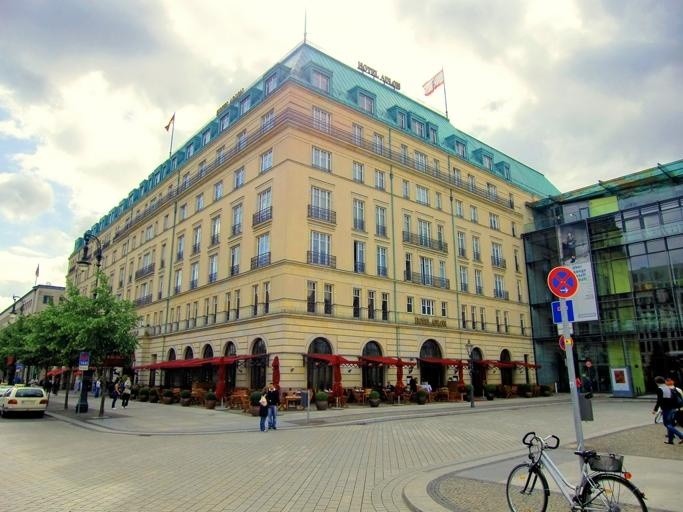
xmin=0 ymin=382 xmax=48 ymax=419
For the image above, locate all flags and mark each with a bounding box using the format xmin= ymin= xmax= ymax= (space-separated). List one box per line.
xmin=163 ymin=113 xmax=174 ymax=131
xmin=422 ymin=69 xmax=443 ymax=97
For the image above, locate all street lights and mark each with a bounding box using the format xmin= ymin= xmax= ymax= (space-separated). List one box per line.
xmin=74 ymin=232 xmax=106 ymax=414
xmin=5 ymin=296 xmax=26 ymax=384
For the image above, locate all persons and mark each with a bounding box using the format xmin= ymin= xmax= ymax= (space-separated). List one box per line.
xmin=664 ymin=377 xmax=683 ymax=428
xmin=583 ymin=376 xmax=593 ymax=391
xmin=10 ymin=370 xmax=81 ymax=399
xmin=93 ymin=376 xmax=101 ymax=397
xmin=386 ymin=381 xmax=394 ymax=391
xmin=576 ymin=375 xmax=581 ymax=393
xmin=109 ymin=377 xmax=121 ymax=408
xmin=648 ymin=376 xmax=683 ymax=445
xmin=266 ymin=383 xmax=279 ymax=430
xmin=258 ymin=386 xmax=269 ymax=432
xmin=120 ymin=375 xmax=132 ymax=409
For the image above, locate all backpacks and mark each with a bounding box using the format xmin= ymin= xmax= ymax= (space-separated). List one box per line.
xmin=671 ymin=388 xmax=683 ymax=409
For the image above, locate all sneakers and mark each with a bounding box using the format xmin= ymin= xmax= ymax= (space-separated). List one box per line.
xmin=663 ymin=434 xmax=683 ymax=446
xmin=261 ymin=427 xmax=276 ymax=432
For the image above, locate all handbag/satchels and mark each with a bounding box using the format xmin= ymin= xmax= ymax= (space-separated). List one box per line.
xmin=259 ymin=394 xmax=268 ymax=407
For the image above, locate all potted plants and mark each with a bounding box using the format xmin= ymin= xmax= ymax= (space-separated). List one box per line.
xmin=131 ymin=387 xmax=216 ymax=409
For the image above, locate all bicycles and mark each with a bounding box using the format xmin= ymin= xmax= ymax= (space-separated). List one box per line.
xmin=502 ymin=431 xmax=649 ymax=511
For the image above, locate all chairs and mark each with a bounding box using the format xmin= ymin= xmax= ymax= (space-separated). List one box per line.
xmin=226 ymin=382 xmax=540 ymax=413
